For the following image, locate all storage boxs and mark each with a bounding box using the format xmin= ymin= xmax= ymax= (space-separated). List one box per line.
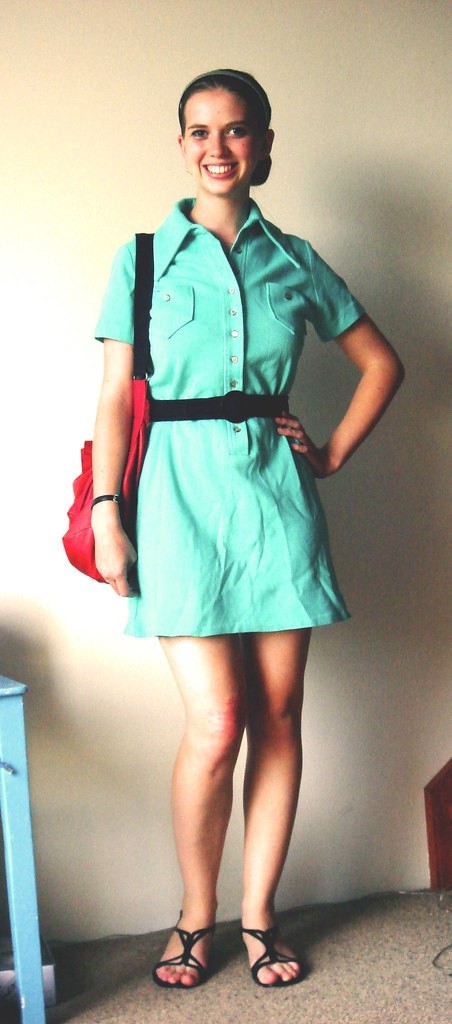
xmin=0 ymin=932 xmax=56 ymax=1011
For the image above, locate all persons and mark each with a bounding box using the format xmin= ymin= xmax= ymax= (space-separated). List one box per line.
xmin=91 ymin=69 xmax=402 ymax=988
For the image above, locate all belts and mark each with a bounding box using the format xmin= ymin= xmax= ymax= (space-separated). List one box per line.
xmin=150 ymin=390 xmax=292 ymax=420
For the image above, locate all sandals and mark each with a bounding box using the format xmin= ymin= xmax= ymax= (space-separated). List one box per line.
xmin=240 ymin=924 xmax=307 ymax=987
xmin=152 ymin=908 xmax=216 ymax=989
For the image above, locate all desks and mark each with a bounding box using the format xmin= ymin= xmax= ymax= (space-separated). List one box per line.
xmin=0 ymin=679 xmax=46 ymax=1024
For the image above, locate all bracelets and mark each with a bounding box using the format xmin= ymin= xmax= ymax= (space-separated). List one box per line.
xmin=91 ymin=494 xmax=124 ymax=507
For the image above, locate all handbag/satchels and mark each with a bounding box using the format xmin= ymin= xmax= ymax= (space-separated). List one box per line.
xmin=62 ymin=378 xmax=151 ymax=585
